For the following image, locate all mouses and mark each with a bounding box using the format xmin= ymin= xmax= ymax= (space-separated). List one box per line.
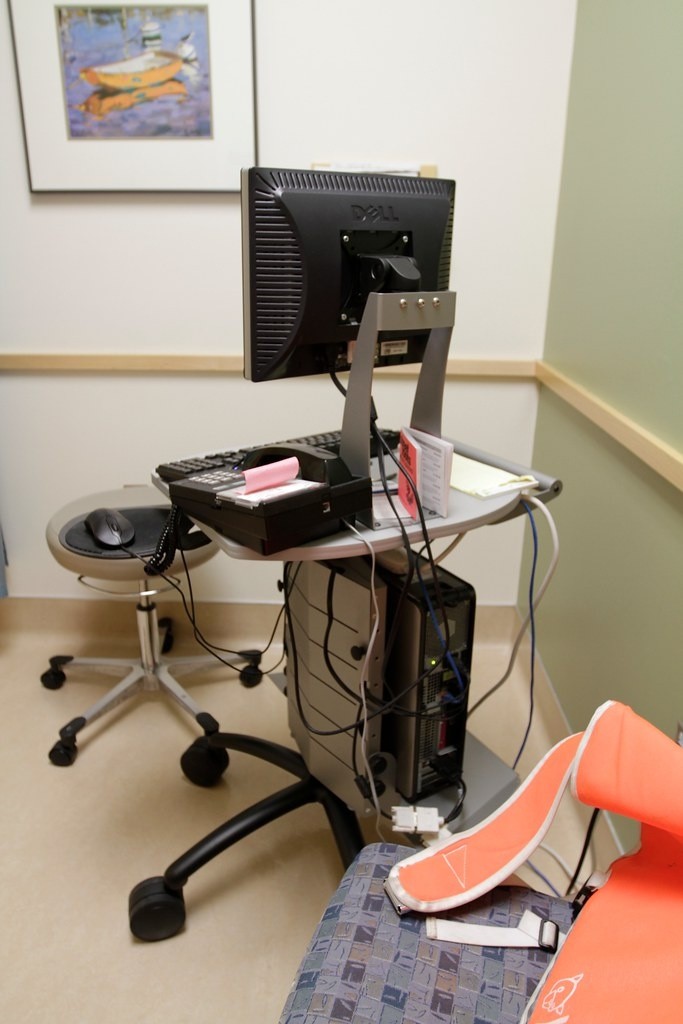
xmin=84 ymin=507 xmax=136 ymax=546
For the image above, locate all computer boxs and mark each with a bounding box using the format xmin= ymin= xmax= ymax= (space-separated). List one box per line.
xmin=283 ymin=547 xmax=476 ymax=803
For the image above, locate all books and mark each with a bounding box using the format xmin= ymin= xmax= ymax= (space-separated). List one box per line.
xmin=399 ymin=425 xmax=454 ymax=522
xmin=449 ymin=452 xmax=538 ymax=501
xmin=357 ymin=479 xmax=412 ymax=530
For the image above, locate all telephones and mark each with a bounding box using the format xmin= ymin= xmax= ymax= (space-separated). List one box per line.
xmin=168 ymin=443 xmax=374 ymax=557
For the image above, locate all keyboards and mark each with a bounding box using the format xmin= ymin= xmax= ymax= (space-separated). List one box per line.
xmin=157 ymin=430 xmax=401 ymax=478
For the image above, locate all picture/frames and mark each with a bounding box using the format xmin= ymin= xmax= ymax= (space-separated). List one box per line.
xmin=7 ymin=0 xmax=257 ymax=194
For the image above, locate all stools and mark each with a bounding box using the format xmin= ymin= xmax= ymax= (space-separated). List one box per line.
xmin=37 ymin=481 xmax=264 ymax=785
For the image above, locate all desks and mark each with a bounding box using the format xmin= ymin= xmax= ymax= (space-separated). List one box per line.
xmin=127 ymin=444 xmax=520 ymax=941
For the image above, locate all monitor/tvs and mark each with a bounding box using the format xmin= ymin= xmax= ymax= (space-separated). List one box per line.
xmin=240 ymin=165 xmax=455 ymax=480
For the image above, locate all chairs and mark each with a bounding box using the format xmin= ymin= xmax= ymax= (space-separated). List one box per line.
xmin=277 ymin=700 xmax=683 ymax=1024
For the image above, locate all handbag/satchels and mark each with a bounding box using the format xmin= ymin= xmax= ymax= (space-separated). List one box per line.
xmin=382 ymin=699 xmax=683 ymax=1024
